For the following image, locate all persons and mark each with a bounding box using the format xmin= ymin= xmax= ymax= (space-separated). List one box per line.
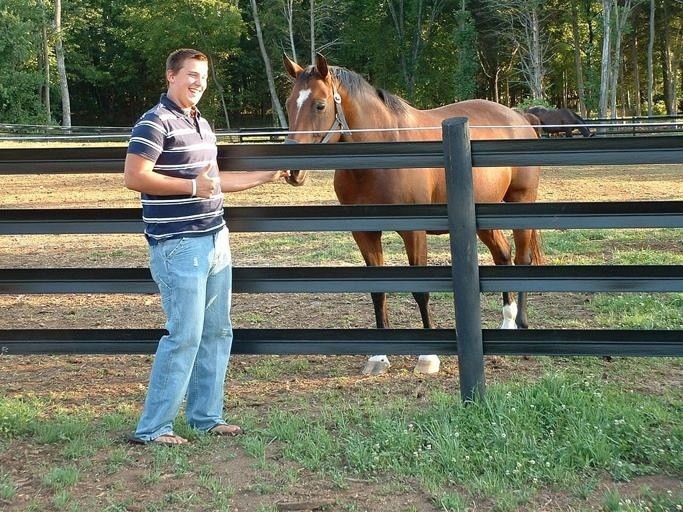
xmin=121 ymin=46 xmax=292 ymax=446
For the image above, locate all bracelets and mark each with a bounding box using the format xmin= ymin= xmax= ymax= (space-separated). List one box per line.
xmin=191 ymin=179 xmax=196 ymax=197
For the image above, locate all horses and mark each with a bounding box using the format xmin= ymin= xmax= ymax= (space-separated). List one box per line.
xmin=526 ymin=105 xmax=596 ymax=139
xmin=280 ymin=51 xmax=546 ymax=377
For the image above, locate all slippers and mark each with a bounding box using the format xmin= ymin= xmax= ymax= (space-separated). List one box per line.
xmin=128 ymin=432 xmax=189 ymax=447
xmin=209 ymin=421 xmax=245 ymax=438
